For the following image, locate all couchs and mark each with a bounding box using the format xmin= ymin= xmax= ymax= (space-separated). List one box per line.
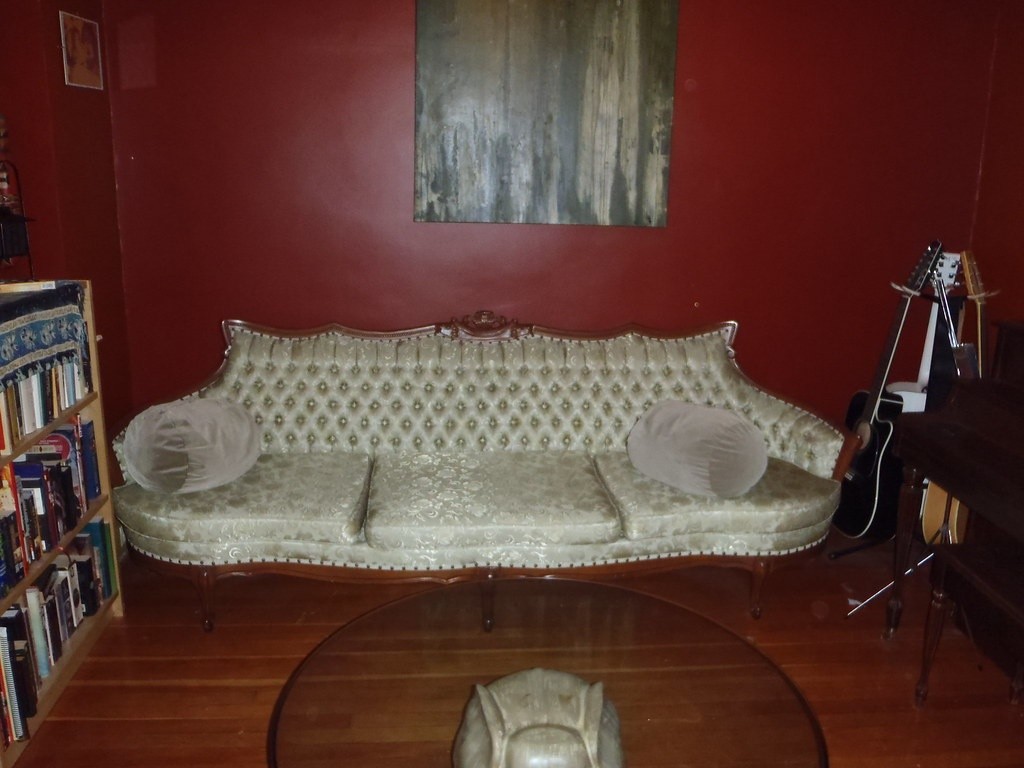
xmin=118 ymin=313 xmax=845 ymax=570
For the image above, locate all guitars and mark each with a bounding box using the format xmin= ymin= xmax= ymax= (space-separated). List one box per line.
xmin=834 ymin=235 xmax=990 ymax=550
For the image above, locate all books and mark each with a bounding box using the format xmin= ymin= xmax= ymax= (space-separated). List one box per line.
xmin=0 ymin=517 xmax=118 ymax=751
xmin=0 ymin=418 xmax=100 ymax=599
xmin=0 ymin=351 xmax=83 ymax=456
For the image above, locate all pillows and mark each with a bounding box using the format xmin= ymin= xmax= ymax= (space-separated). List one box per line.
xmin=119 ymin=398 xmax=265 ymax=488
xmin=624 ymin=400 xmax=769 ymax=496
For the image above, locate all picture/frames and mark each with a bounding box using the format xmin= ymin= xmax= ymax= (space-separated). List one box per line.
xmin=59 ymin=12 xmax=105 ymax=90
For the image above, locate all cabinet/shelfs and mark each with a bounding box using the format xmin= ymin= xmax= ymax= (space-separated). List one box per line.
xmin=0 ymin=279 xmax=126 ymax=768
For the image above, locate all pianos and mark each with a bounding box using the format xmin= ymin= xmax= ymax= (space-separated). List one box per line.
xmin=884 ymin=315 xmax=1024 ymax=704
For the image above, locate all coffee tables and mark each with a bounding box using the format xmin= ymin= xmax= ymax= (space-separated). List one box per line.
xmin=271 ymin=576 xmax=828 ymax=768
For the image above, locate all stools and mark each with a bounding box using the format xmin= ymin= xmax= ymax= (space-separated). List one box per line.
xmin=914 ymin=538 xmax=1024 ymax=714
